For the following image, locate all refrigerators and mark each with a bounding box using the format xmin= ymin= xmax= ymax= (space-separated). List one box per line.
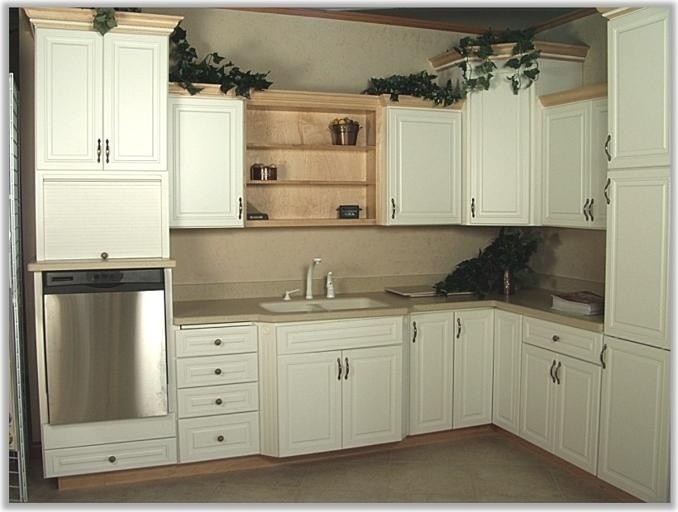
xmin=44 ymin=270 xmax=168 ymax=427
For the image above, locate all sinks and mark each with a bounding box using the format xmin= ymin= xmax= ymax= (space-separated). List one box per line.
xmin=322 ymin=297 xmax=389 ymax=309
xmin=259 ymin=300 xmax=321 ymax=313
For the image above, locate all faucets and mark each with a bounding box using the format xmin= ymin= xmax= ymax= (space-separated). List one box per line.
xmin=326 ymin=272 xmax=337 ymax=298
xmin=306 ymin=257 xmax=322 ymax=299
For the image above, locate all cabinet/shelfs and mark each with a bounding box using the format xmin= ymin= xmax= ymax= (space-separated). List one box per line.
xmin=596 ymin=6 xmax=672 ymax=169
xmin=266 ymin=316 xmax=403 ymax=458
xmin=243 ymin=88 xmax=379 ymax=229
xmin=431 ymin=42 xmax=590 ymax=225
xmin=493 ymin=309 xmax=669 ymax=502
xmin=168 ymin=82 xmax=244 ymax=229
xmin=380 ymin=93 xmax=464 ymax=226
xmin=411 ymin=308 xmax=495 ymax=436
xmin=541 ymin=82 xmax=607 ymax=230
xmin=603 ymin=169 xmax=670 ymax=350
xmin=23 ymin=7 xmax=183 ymax=170
xmin=174 ymin=322 xmax=266 ymax=465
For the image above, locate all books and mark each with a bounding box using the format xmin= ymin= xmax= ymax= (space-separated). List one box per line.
xmin=386 ymin=285 xmax=476 ymax=298
xmin=550 ymin=290 xmax=604 ymax=316
